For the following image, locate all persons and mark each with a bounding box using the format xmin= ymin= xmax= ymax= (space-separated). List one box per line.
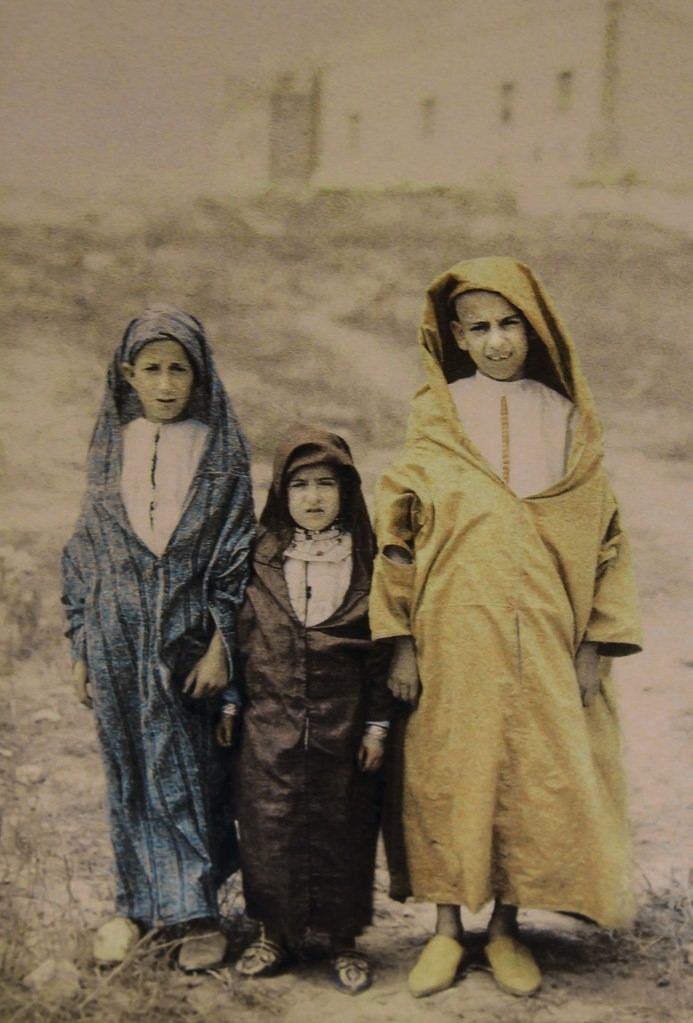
xmin=62 ymin=308 xmax=257 ymax=969
xmin=369 ymin=254 xmax=641 ymax=996
xmin=218 ymin=431 xmax=393 ymax=992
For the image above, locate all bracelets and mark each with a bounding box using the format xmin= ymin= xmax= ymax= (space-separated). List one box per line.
xmin=368 ymin=726 xmax=389 ymax=738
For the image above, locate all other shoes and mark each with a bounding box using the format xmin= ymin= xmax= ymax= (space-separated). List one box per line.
xmin=236 ymin=941 xmax=288 ymax=977
xmin=93 ymin=916 xmax=140 ymax=967
xmin=484 ymin=936 xmax=541 ymax=996
xmin=409 ymin=931 xmax=466 ymax=996
xmin=179 ymin=923 xmax=226 ymax=971
xmin=331 ymin=948 xmax=370 ymax=996
